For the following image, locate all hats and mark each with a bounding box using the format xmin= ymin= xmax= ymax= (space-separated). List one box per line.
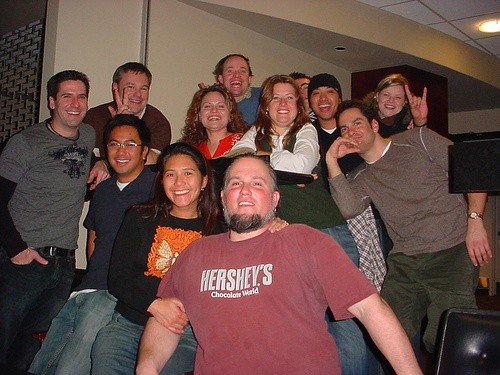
xmin=307 ymin=73 xmax=342 ymax=99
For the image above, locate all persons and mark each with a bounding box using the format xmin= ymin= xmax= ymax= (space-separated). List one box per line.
xmin=0 ymin=52 xmax=493 ymax=375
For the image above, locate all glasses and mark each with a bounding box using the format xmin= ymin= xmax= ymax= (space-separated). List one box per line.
xmin=106 ymin=140 xmax=143 ymax=149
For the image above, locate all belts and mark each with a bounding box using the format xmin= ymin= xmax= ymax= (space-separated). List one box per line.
xmin=34 ymin=246 xmax=75 ymax=258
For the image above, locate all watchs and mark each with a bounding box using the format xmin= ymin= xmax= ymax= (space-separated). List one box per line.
xmin=468 ymin=211 xmax=483 ymax=220
